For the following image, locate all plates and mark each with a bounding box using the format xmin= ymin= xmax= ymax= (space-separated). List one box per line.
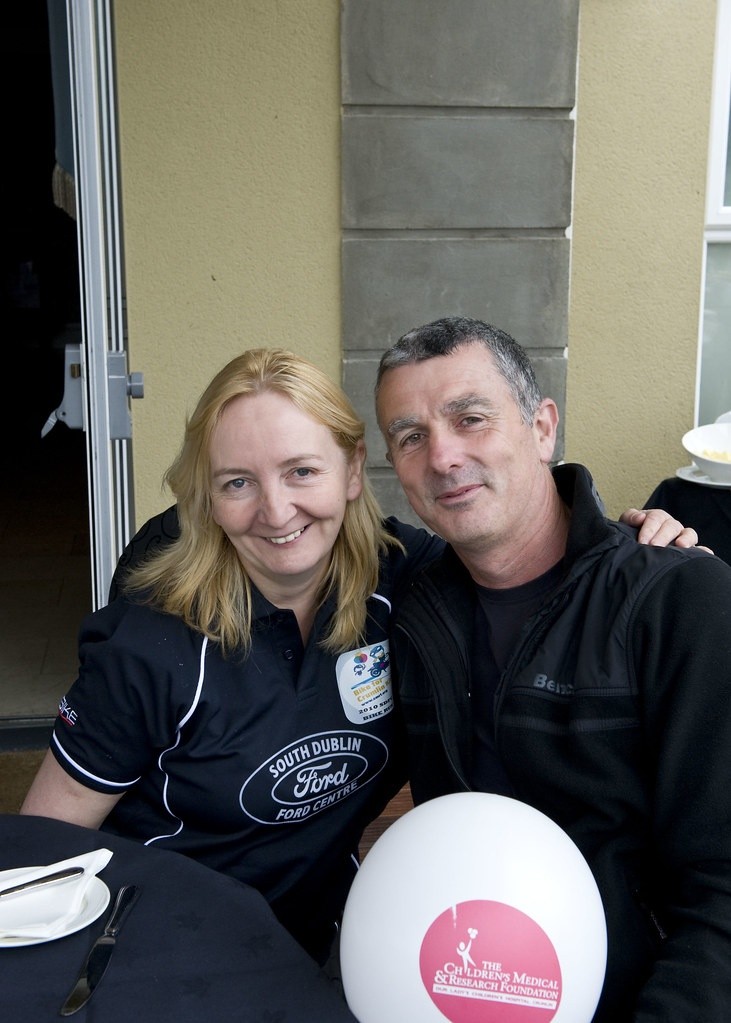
xmin=676 ymin=465 xmax=731 ymax=486
xmin=0 ymin=867 xmax=110 ymax=948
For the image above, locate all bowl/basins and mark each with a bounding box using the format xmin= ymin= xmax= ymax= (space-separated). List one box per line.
xmin=683 ymin=423 xmax=731 ymax=479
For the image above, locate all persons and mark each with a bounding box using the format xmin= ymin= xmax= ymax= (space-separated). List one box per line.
xmin=375 ymin=316 xmax=731 ymax=1023
xmin=20 ymin=350 xmax=712 ymax=968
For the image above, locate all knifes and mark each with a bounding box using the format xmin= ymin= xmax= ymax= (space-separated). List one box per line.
xmin=60 ymin=884 xmax=143 ymax=1017
